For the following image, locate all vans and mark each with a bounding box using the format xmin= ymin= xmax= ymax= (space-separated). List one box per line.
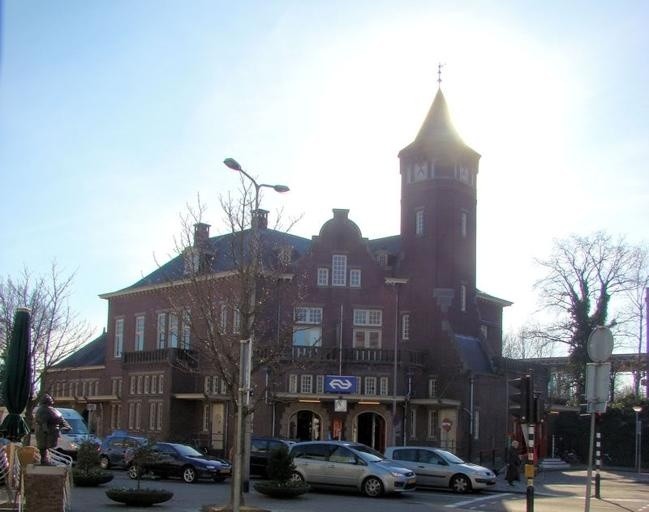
xmin=31 ymin=406 xmax=102 ymax=466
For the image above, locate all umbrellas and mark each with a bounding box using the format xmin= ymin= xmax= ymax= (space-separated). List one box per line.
xmin=0 ymin=306 xmax=33 ymax=487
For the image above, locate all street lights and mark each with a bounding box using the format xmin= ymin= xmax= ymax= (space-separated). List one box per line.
xmin=223 ymin=157 xmax=291 ymax=494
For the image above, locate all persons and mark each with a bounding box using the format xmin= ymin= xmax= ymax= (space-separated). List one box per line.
xmin=555 ymin=436 xmax=564 ymax=461
xmin=34 ymin=393 xmax=72 ymax=466
xmin=504 ymin=440 xmax=522 ymax=487
xmin=0 ymin=445 xmax=42 ymax=465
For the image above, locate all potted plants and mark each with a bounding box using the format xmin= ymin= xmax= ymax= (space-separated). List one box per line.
xmin=253 ymin=445 xmax=311 ymax=500
xmin=73 ymin=435 xmax=173 ymax=506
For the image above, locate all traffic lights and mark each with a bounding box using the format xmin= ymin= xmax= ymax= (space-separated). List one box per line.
xmin=507 ymin=373 xmax=536 ymax=424
